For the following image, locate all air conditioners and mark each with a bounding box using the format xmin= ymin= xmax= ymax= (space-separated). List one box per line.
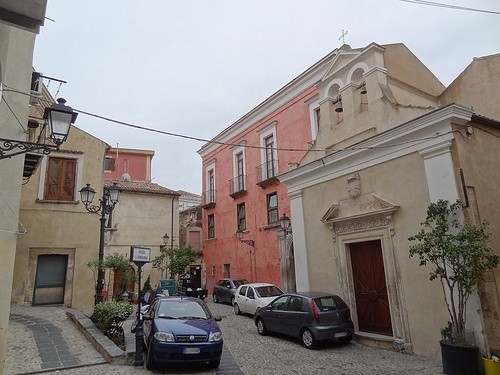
xmin=30 ymin=71 xmax=43 ymax=105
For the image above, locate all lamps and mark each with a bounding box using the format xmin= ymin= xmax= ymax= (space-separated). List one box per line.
xmin=160 ymin=233 xmax=169 ymax=249
xmin=235 ymin=228 xmax=255 ymax=248
xmin=0 ymin=97 xmax=79 ymax=161
xmin=278 ymin=213 xmax=292 ymax=235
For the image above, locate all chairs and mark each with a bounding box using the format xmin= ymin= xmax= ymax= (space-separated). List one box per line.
xmin=234 ymin=281 xmax=240 ymax=288
xmin=292 ymin=299 xmax=300 ymax=311
xmin=227 ymin=281 xmax=231 ymax=287
xmin=261 ymin=288 xmax=270 ymax=295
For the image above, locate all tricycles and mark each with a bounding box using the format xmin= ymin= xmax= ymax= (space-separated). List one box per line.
xmin=176 ymin=277 xmax=209 ymax=301
xmin=154 ymin=278 xmax=176 ymax=296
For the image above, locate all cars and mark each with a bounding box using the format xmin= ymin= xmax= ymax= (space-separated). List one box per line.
xmin=232 ymin=281 xmax=287 ymax=318
xmin=141 ymin=295 xmax=224 ymax=372
xmin=251 ymin=289 xmax=356 ymax=352
xmin=212 ymin=278 xmax=251 ymax=306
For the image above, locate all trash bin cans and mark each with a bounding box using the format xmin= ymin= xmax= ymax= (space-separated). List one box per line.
xmin=482 ymin=356 xmax=500 ymax=375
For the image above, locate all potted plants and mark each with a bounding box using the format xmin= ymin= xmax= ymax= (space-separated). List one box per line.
xmin=408 ymin=197 xmax=500 ymax=375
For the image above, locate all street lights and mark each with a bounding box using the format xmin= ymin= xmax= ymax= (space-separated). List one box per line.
xmin=162 ymin=233 xmax=170 ymax=279
xmin=78 ymin=181 xmax=123 ymax=306
